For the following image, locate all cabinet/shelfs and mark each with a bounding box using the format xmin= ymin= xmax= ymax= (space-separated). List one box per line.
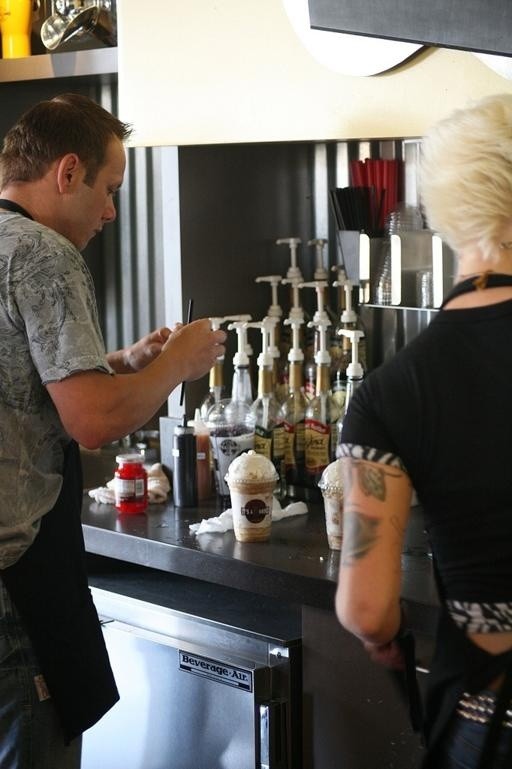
xmin=2 ymin=0 xmax=512 ymax=768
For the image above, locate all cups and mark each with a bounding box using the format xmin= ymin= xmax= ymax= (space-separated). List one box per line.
xmin=317 ymin=459 xmax=350 ymax=548
xmin=226 ymin=453 xmax=279 ymax=543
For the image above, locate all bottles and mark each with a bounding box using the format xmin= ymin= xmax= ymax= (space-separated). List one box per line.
xmin=115 ymin=355 xmax=363 ymax=514
xmin=0 ymin=0 xmax=32 ymax=58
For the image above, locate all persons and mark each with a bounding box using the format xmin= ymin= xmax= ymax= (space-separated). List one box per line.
xmin=331 ymin=93 xmax=511 ymax=769
xmin=2 ymin=91 xmax=231 ymax=769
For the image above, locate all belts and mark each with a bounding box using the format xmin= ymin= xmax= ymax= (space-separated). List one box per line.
xmin=455 ymin=690 xmax=512 ymax=730
